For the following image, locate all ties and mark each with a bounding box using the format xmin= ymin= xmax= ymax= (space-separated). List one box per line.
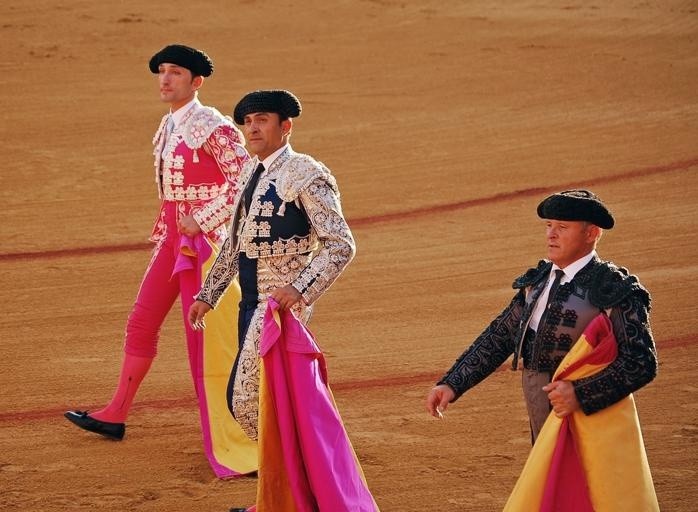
xmin=245 ymin=163 xmax=264 ymax=216
xmin=548 ymin=270 xmax=565 ymax=305
xmin=161 ymin=116 xmax=174 ymax=161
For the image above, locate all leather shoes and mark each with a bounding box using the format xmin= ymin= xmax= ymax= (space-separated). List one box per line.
xmin=63 ymin=410 xmax=125 ymax=441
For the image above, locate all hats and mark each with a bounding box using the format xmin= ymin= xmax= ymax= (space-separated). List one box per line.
xmin=234 ymin=91 xmax=302 ymax=125
xmin=149 ymin=44 xmax=213 ymax=78
xmin=538 ymin=189 xmax=615 ymax=229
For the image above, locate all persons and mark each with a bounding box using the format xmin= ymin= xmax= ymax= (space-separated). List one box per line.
xmin=187 ymin=90 xmax=380 ymax=511
xmin=426 ymin=189 xmax=661 ymax=450
xmin=63 ymin=45 xmax=253 ymax=440
